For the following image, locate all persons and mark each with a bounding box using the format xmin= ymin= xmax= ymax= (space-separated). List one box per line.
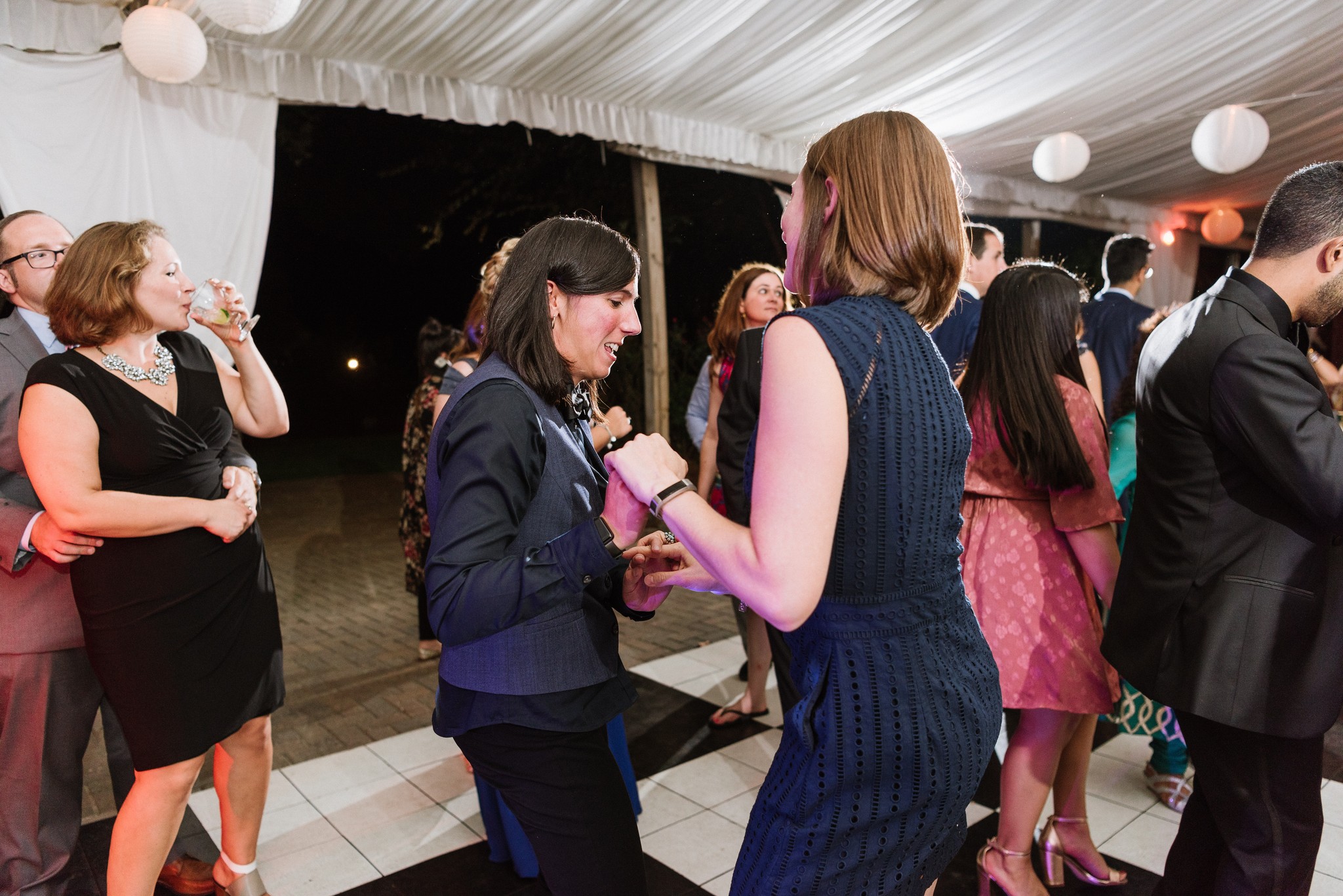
xmin=17 ymin=221 xmax=290 ymax=895
xmin=423 ymin=215 xmax=679 ymax=896
xmin=407 ymin=321 xmax=467 ymax=658
xmin=0 ymin=212 xmax=227 ymax=896
xmin=602 ymin=112 xmax=1001 ymax=895
xmin=685 ymin=264 xmax=785 ymax=729
xmin=432 ymin=238 xmax=523 ymax=433
xmin=1305 ymin=348 xmax=1340 ymax=386
xmin=1095 ymin=162 xmax=1343 ymax=896
xmin=915 ymin=220 xmax=1200 ymax=896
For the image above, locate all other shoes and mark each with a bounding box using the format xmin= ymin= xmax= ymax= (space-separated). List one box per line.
xmin=742 ymin=655 xmax=773 ymax=680
xmin=418 ymin=649 xmax=443 ymax=659
xmin=1144 ymin=764 xmax=1194 ymax=812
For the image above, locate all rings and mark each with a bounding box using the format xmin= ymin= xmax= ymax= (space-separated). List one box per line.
xmin=664 ymin=532 xmax=675 ymax=545
xmin=244 ymin=503 xmax=252 ymax=510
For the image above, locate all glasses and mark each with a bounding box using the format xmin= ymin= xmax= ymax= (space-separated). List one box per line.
xmin=0 ymin=245 xmax=71 ymax=272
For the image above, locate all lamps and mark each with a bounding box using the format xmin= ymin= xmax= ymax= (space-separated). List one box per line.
xmin=118 ymin=3 xmax=207 ymax=88
xmin=1198 ymin=206 xmax=1246 ymax=247
xmin=1031 ymin=129 xmax=1092 ymax=185
xmin=195 ymin=0 xmax=303 ymax=37
xmin=1188 ymin=102 xmax=1272 ymax=177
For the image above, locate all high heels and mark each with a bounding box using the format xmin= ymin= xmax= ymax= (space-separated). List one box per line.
xmin=1036 ymin=814 xmax=1129 ymax=886
xmin=211 ymin=853 xmax=273 ymax=896
xmin=974 ymin=834 xmax=1048 ymax=896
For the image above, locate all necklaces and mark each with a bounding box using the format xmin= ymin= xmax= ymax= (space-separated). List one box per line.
xmin=97 ymin=344 xmax=176 ymax=385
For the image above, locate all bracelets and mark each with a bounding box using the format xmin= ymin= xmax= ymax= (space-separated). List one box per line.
xmin=1308 ymin=351 xmax=1319 ymax=363
xmin=648 ymin=478 xmax=698 ymax=520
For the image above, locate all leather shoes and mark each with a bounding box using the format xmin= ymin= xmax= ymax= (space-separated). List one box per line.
xmin=154 ymin=854 xmax=215 ymax=894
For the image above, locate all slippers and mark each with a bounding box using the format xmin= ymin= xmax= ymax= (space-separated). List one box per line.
xmin=709 ymin=702 xmax=769 ymax=727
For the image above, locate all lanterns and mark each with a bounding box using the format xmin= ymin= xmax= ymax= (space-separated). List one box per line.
xmin=1191 ymin=105 xmax=1270 ymax=174
xmin=1032 ymin=132 xmax=1090 ymax=182
xmin=1201 ymin=209 xmax=1243 ymax=245
xmin=202 ymin=1 xmax=300 ymax=34
xmin=121 ymin=6 xmax=208 ymax=85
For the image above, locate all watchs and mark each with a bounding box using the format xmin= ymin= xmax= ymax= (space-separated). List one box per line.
xmin=239 ymin=466 xmax=261 ymax=493
xmin=595 ymin=516 xmax=624 ymax=560
